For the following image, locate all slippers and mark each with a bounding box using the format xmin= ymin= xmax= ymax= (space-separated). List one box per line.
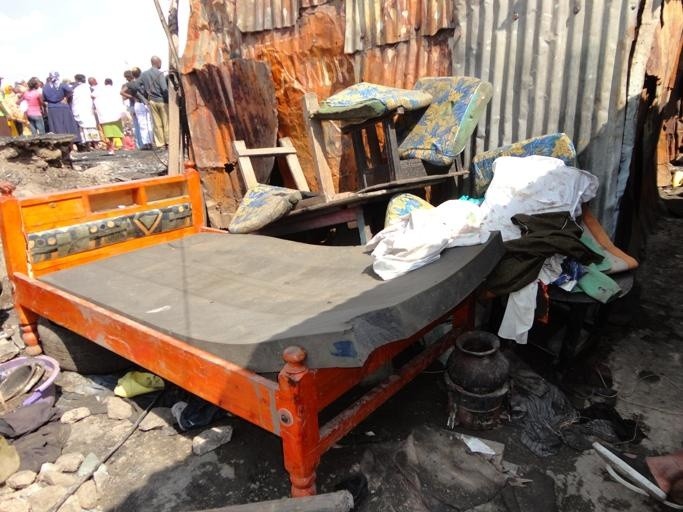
xmin=593 ymin=441 xmax=681 ymax=509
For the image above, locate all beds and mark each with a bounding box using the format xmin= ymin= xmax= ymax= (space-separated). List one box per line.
xmin=0 ymin=161 xmax=478 ymax=497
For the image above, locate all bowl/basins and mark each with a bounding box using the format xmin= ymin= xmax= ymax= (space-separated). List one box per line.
xmin=0 ymin=354 xmax=60 ymax=408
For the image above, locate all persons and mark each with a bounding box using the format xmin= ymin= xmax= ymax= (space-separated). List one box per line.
xmin=0 ymin=56 xmax=169 ymax=155
